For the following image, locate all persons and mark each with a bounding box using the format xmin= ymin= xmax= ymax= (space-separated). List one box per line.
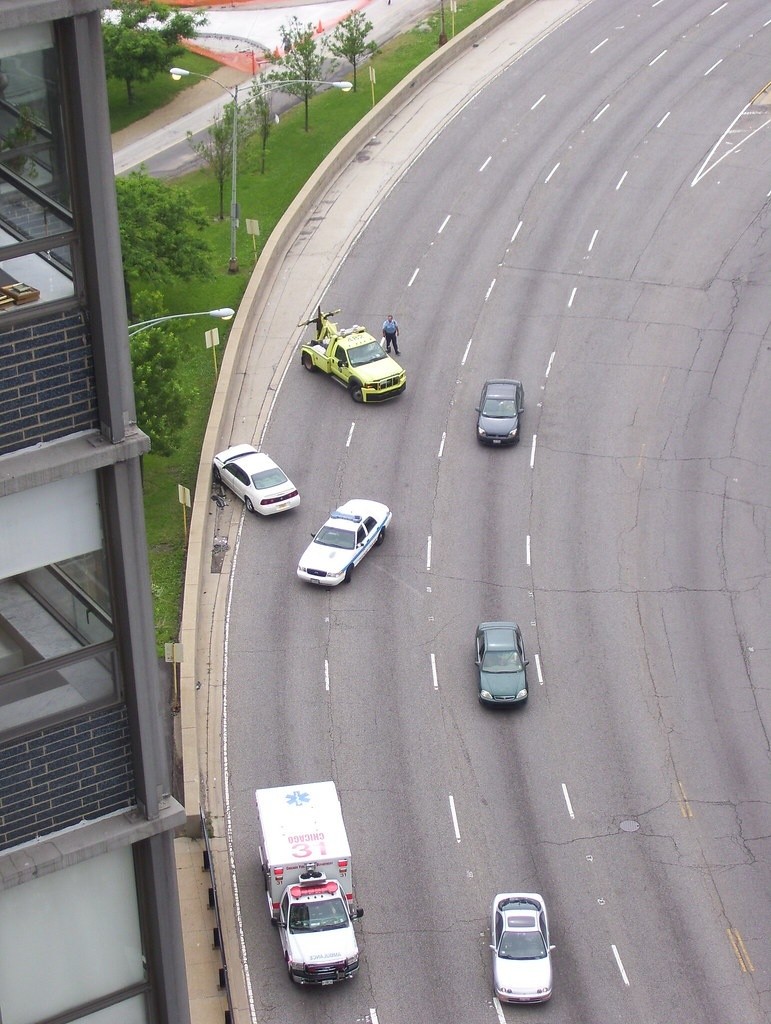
xmin=382 ymin=315 xmax=399 ymax=355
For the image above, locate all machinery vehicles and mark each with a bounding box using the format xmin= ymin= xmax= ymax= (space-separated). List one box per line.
xmin=297 ymin=305 xmax=406 ymax=404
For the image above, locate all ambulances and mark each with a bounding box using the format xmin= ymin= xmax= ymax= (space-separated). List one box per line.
xmin=254 ymin=780 xmax=365 ymax=988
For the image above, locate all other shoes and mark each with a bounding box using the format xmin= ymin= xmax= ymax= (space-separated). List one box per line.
xmin=396 ymin=351 xmax=401 ymax=355
xmin=386 ymin=349 xmax=391 ymax=353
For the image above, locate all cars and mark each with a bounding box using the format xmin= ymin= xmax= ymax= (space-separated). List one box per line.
xmin=476 ymin=378 xmax=525 ymax=445
xmin=214 ymin=443 xmax=301 ymax=516
xmin=490 ymin=892 xmax=556 ymax=1005
xmin=296 ymin=498 xmax=392 ymax=588
xmin=475 ymin=621 xmax=529 ymax=708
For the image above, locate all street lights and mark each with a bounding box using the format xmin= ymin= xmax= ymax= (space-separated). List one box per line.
xmin=171 ymin=67 xmax=353 ymax=272
